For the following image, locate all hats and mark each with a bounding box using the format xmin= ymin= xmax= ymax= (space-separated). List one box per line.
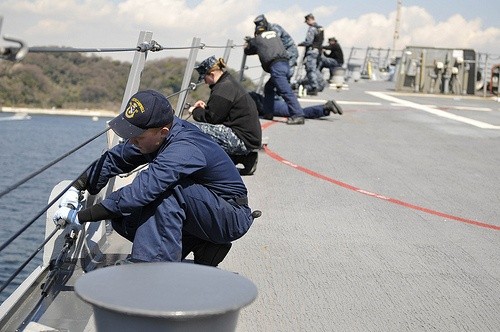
xmin=108 ymin=90 xmax=174 ymax=139
xmin=255 ymin=26 xmax=266 ymax=33
xmin=194 ymin=55 xmax=217 ymax=83
xmin=254 ymin=14 xmax=266 ymax=26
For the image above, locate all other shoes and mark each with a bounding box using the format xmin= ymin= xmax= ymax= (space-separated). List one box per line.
xmin=262 ymin=113 xmax=273 ymax=120
xmin=326 ymin=100 xmax=343 ymax=114
xmin=243 ymin=152 xmax=258 ymax=175
xmin=287 ymin=117 xmax=304 ymax=124
xmin=194 ymin=243 xmax=232 ymax=267
xmin=307 ymin=89 xmax=317 ymax=95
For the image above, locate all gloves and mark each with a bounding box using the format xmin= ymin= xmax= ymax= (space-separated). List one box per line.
xmin=244 ymin=36 xmax=252 ymax=43
xmin=53 ymin=206 xmax=81 ymax=226
xmin=58 ymin=186 xmax=80 ymax=210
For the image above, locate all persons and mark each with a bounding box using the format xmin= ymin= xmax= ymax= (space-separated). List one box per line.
xmin=53 ymin=14 xmax=344 ymax=295
